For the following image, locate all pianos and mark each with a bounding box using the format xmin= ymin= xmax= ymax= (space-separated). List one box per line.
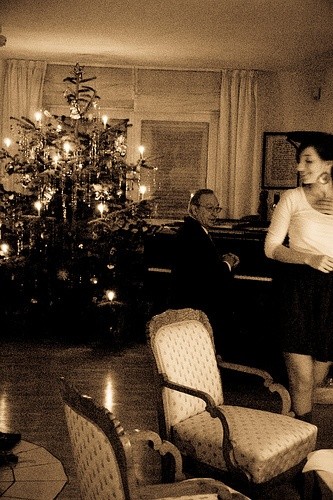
xmin=142 ymin=218 xmax=333 ymax=372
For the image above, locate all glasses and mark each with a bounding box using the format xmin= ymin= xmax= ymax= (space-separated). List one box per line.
xmin=195 ymin=204 xmax=222 ymax=213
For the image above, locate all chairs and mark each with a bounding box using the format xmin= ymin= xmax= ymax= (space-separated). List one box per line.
xmin=145 ymin=307 xmax=319 ymax=500
xmin=58 ymin=376 xmax=247 ymax=500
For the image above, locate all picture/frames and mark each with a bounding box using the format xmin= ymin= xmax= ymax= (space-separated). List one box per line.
xmin=261 ymin=130 xmax=301 ymax=190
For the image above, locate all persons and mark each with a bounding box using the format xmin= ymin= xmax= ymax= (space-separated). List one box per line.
xmin=167 ymin=189 xmax=240 ymax=360
xmin=262 ymin=134 xmax=333 ymax=425
